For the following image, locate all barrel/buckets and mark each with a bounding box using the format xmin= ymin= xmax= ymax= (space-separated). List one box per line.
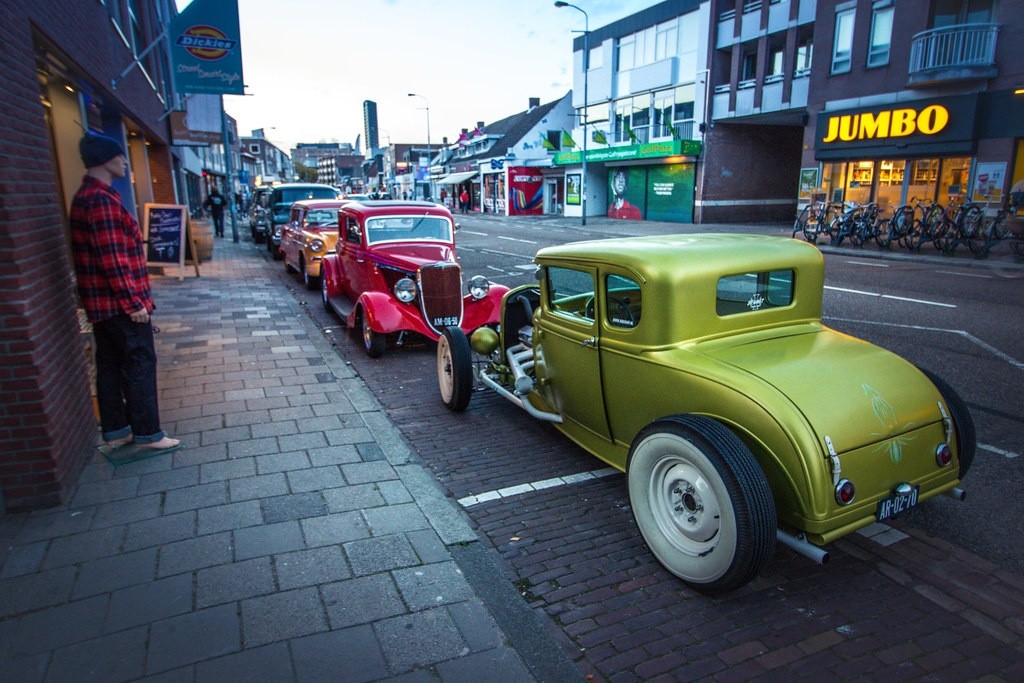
xmin=188 ymin=220 xmax=213 ymax=262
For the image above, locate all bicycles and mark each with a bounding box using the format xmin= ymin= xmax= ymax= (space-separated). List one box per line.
xmin=803 ymin=194 xmax=1015 ymax=259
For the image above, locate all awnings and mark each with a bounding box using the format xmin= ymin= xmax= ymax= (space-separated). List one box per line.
xmin=436 ymin=171 xmax=478 ymax=184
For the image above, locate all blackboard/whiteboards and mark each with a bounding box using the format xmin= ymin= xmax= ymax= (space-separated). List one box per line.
xmin=143 ymin=202 xmax=187 ymax=268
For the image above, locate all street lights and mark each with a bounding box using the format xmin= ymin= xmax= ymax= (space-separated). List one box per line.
xmin=408 ymin=94 xmax=432 ymax=197
xmin=554 ymin=1 xmax=588 ymax=229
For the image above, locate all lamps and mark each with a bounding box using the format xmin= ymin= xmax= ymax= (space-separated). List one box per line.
xmin=801 ymin=112 xmax=809 ymax=126
xmin=698 ymin=123 xmax=706 ymax=133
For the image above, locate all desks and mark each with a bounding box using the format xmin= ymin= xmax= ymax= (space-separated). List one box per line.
xmin=945 ymin=194 xmax=965 ymax=207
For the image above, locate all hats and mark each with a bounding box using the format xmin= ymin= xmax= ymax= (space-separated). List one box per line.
xmin=79 ymin=134 xmax=124 ymax=168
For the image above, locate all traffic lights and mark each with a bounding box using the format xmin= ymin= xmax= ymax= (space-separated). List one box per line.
xmin=202 ymin=168 xmax=208 ymax=176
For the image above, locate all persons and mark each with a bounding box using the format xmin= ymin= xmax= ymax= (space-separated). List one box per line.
xmin=440 ymin=188 xmax=446 ymax=202
xmin=70 ymin=133 xmax=180 ymax=449
xmin=403 ymin=189 xmax=413 ymax=200
xmin=203 ymin=188 xmax=227 ymax=238
xmin=460 ymin=190 xmax=468 ymax=214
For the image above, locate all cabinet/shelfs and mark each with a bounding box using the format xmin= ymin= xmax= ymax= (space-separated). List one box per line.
xmin=853 ymin=160 xmax=939 ymax=188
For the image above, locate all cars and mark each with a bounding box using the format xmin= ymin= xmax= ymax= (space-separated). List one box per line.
xmin=437 ymin=234 xmax=976 ymax=594
xmin=320 ymin=200 xmax=513 ymax=358
xmin=278 ymin=200 xmax=357 ymax=291
xmin=251 ymin=182 xmax=392 ymax=260
